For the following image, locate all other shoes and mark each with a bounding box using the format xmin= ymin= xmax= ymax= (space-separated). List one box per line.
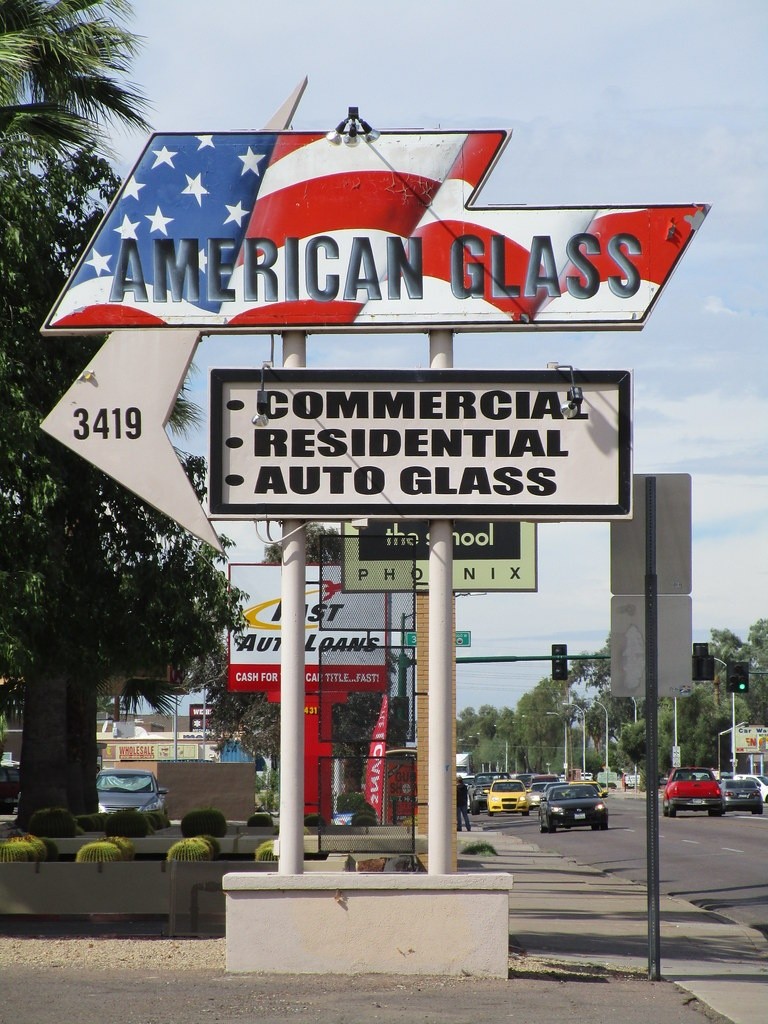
xmin=468 ymin=829 xmax=471 ymax=831
xmin=457 ymin=828 xmax=462 ymax=831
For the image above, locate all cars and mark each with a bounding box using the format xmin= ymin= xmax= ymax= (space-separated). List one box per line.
xmin=97 ymin=768 xmax=169 ymax=818
xmin=537 ymin=785 xmax=609 ymax=834
xmin=511 ymin=774 xmax=560 ymax=791
xmin=483 ymin=779 xmax=533 ymax=817
xmin=564 ymin=781 xmax=608 ymax=801
xmin=559 ymin=774 xmax=565 ymax=783
xmin=1 ymin=766 xmax=21 ymax=815
xmin=526 ymin=782 xmax=550 ymax=809
xmin=539 ymin=783 xmax=568 ymax=804
xmin=462 ymin=777 xmax=484 ymax=810
xmin=718 ymin=778 xmax=763 ymax=815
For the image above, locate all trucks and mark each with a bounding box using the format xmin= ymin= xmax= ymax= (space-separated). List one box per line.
xmin=456 ymin=753 xmax=472 ymax=779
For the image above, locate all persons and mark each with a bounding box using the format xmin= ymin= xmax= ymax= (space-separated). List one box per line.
xmin=457 ymin=777 xmax=471 ymax=832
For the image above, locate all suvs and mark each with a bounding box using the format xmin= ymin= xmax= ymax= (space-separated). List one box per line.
xmin=581 ymin=773 xmax=594 ymax=781
xmin=467 ymin=773 xmax=513 ymax=815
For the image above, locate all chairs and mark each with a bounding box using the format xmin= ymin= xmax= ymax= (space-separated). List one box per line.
xmin=555 ymin=793 xmax=563 ymax=799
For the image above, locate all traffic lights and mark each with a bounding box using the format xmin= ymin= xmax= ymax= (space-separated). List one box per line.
xmin=726 ymin=661 xmax=749 ymax=693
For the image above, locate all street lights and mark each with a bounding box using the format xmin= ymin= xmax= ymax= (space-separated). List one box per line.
xmin=609 ymin=689 xmax=637 ymax=792
xmin=396 ymin=591 xmax=487 ymax=747
xmin=585 ymin=700 xmax=609 ymax=792
xmin=477 ymin=733 xmax=491 ymax=773
xmin=563 ymin=703 xmax=585 ymax=772
xmin=547 ymin=712 xmax=568 ymax=779
xmin=521 ymin=717 xmax=541 ymax=728
xmin=469 ymin=736 xmax=484 ymax=773
xmin=494 ymin=725 xmax=508 ymax=772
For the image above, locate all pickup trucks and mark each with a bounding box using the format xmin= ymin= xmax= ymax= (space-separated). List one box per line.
xmin=660 ymin=767 xmax=722 ymax=818
xmin=724 ymin=775 xmax=768 ymax=803
